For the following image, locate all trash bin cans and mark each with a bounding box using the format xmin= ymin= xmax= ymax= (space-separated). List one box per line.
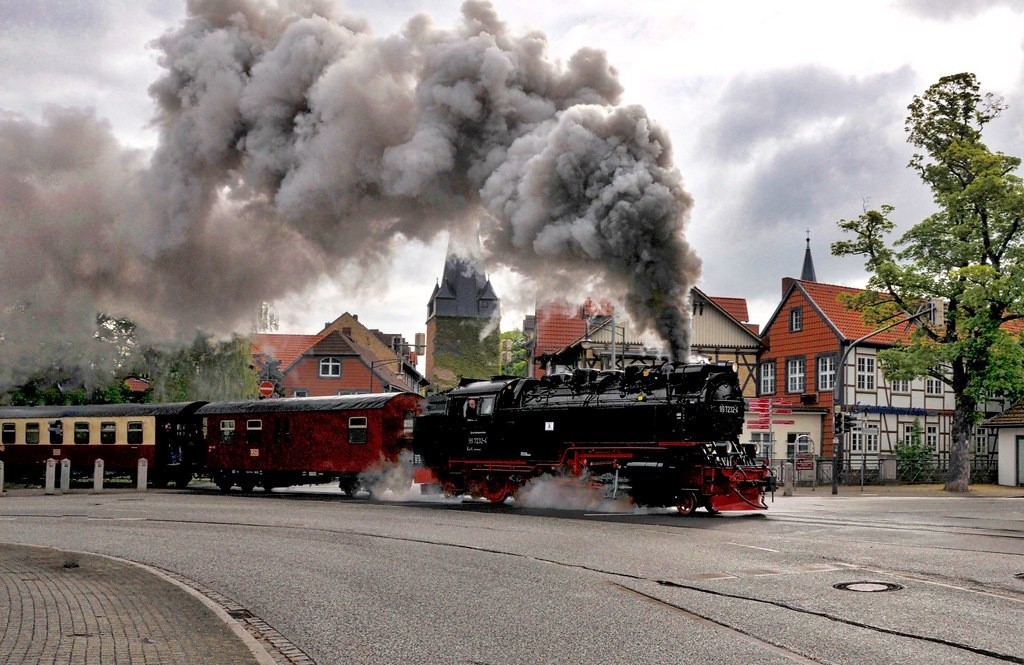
xmin=882 ymin=459 xmax=897 ymax=480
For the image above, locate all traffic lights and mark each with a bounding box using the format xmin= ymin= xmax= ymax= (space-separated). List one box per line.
xmin=844 ymin=414 xmax=856 ymax=432
xmin=835 ymin=412 xmax=843 ymax=434
xmin=934 ymin=297 xmax=949 ymax=324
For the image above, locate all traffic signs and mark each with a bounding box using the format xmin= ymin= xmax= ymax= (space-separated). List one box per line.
xmin=746 ymin=398 xmax=794 ymax=430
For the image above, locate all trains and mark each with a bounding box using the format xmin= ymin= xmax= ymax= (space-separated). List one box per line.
xmin=0 ymin=341 xmax=778 ymax=517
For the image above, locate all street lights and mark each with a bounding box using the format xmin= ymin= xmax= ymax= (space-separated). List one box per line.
xmin=370 ymin=358 xmax=404 ymax=393
xmin=582 ymin=321 xmax=625 ymax=370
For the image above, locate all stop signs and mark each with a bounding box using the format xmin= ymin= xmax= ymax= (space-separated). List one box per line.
xmin=260 ymin=381 xmax=274 ymax=396
